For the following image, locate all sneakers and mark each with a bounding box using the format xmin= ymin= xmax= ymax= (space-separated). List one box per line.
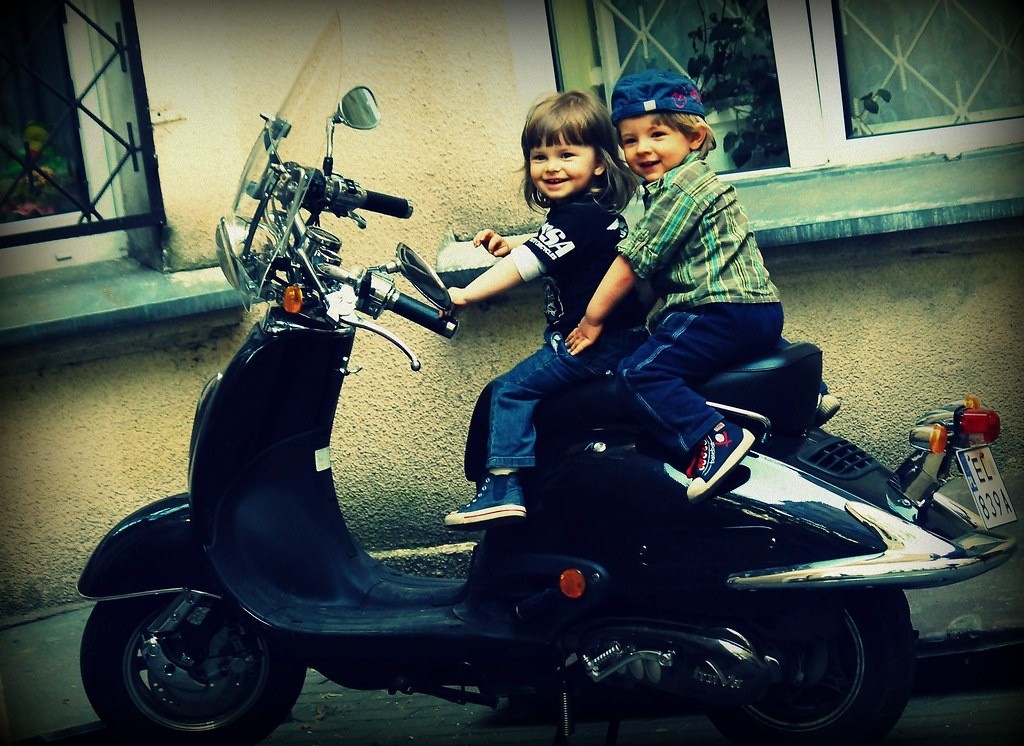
xmin=685 ymin=420 xmax=756 ymax=503
xmin=444 ymin=470 xmax=528 ymax=528
xmin=812 ymin=394 xmax=840 ymax=429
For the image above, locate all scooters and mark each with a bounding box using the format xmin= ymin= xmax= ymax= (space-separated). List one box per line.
xmin=76 ymin=86 xmax=1017 ymax=745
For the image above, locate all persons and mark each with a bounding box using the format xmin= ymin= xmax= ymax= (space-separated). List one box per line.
xmin=445 ymin=91 xmax=655 ymax=526
xmin=565 ymin=69 xmax=784 ymax=500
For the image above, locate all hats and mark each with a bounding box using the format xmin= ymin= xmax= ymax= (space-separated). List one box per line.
xmin=610 ymin=68 xmax=706 ymax=121
